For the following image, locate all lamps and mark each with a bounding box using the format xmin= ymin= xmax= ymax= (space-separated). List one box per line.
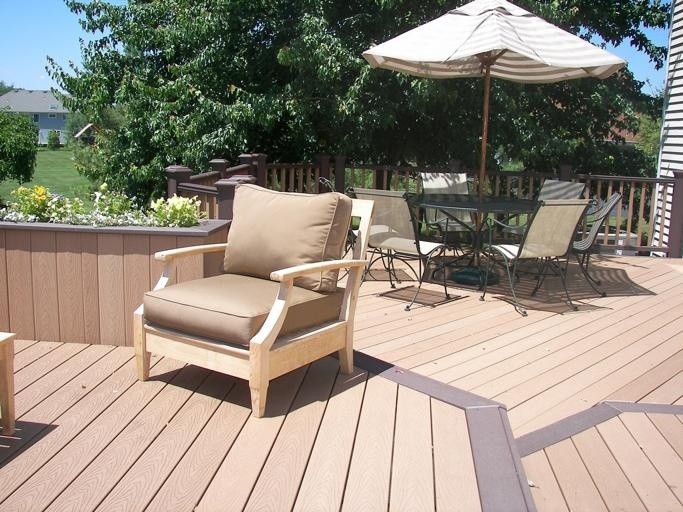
xmin=132 ymin=182 xmax=375 ymax=418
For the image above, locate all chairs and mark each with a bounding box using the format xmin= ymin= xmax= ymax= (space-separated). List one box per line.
xmin=530 ymin=191 xmax=620 ymax=296
xmin=407 ymin=172 xmax=586 ymax=286
xmin=345 ymin=186 xmax=451 ymax=311
xmin=480 ymin=199 xmax=596 ymax=314
xmin=132 ymin=182 xmax=375 ymax=418
xmin=319 ymin=177 xmax=402 ymax=283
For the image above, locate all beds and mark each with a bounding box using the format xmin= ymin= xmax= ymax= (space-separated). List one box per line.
xmin=361 ymin=0 xmax=627 ymax=264
